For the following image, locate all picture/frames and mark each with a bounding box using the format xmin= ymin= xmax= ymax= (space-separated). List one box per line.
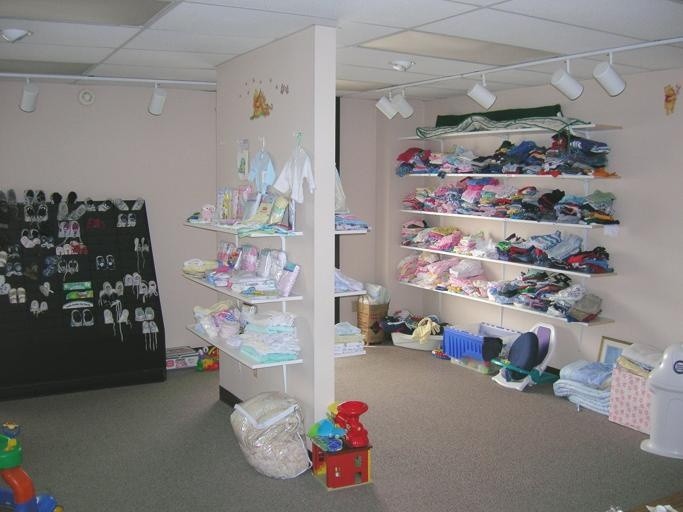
xmin=597 ymin=336 xmax=632 ymax=363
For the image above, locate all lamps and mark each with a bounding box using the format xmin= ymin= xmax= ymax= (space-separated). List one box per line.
xmin=551 ymin=52 xmax=627 ymax=100
xmin=16 ymin=76 xmax=39 ymax=113
xmin=467 ymin=73 xmax=498 ymax=110
xmin=373 ymin=89 xmax=415 ymax=119
xmin=147 ymin=82 xmax=168 ymax=116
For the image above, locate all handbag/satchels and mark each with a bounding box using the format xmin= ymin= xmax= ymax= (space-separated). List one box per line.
xmin=378 ymin=308 xmax=441 ymax=344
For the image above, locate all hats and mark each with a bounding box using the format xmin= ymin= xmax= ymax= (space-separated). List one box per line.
xmin=394 ymin=133 xmax=618 ymax=179
xmin=399 ymin=217 xmax=614 ymax=274
xmin=396 ymin=251 xmax=603 ymax=325
xmin=399 ymin=176 xmax=619 ymax=225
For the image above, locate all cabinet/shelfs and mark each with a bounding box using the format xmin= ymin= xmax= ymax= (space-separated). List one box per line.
xmin=181 ymin=215 xmax=371 ymax=371
xmin=396 ymin=125 xmax=621 ymax=325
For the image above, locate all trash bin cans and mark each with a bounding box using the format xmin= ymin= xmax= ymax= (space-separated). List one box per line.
xmin=640 ymin=343 xmax=683 ymax=459
xmin=358 ymin=300 xmax=389 ymax=345
